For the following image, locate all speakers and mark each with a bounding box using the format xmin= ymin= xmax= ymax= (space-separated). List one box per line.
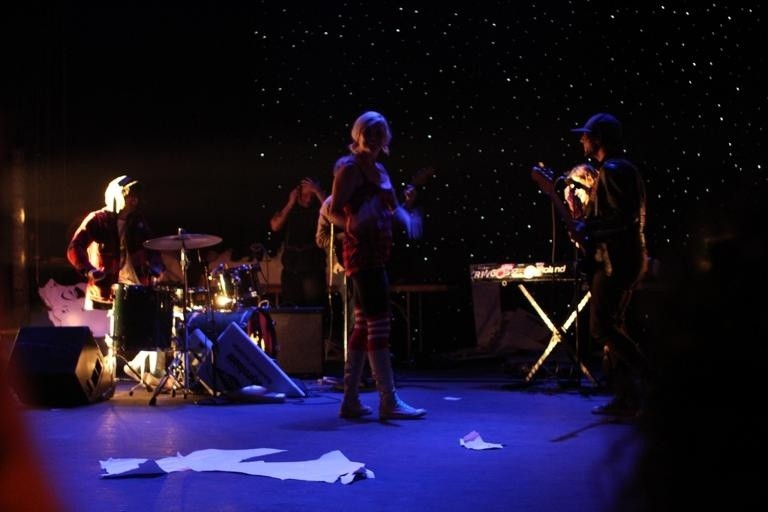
xmin=197 ymin=321 xmax=306 ymax=398
xmin=8 ymin=327 xmax=116 ymax=408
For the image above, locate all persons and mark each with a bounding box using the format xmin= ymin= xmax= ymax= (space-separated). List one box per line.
xmin=532 ymin=114 xmax=650 ymax=414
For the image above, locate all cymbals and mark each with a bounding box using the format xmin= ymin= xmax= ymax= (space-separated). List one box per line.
xmin=143 ymin=234 xmax=222 ymax=250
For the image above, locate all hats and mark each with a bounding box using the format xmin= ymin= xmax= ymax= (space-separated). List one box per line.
xmin=572 ymin=113 xmax=624 ymax=139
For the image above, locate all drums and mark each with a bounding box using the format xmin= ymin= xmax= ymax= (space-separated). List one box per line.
xmin=114 ymin=283 xmax=173 ymax=351
xmin=218 ymin=265 xmax=263 ymax=302
xmin=186 ymin=307 xmax=280 ymax=365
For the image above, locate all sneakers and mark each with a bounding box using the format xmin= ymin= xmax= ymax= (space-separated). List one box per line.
xmin=339 ymin=395 xmax=373 ymax=417
xmin=378 ymin=391 xmax=428 ymax=418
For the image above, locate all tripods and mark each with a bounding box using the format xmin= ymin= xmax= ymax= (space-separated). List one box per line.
xmin=147 ymin=245 xmax=216 ymax=406
